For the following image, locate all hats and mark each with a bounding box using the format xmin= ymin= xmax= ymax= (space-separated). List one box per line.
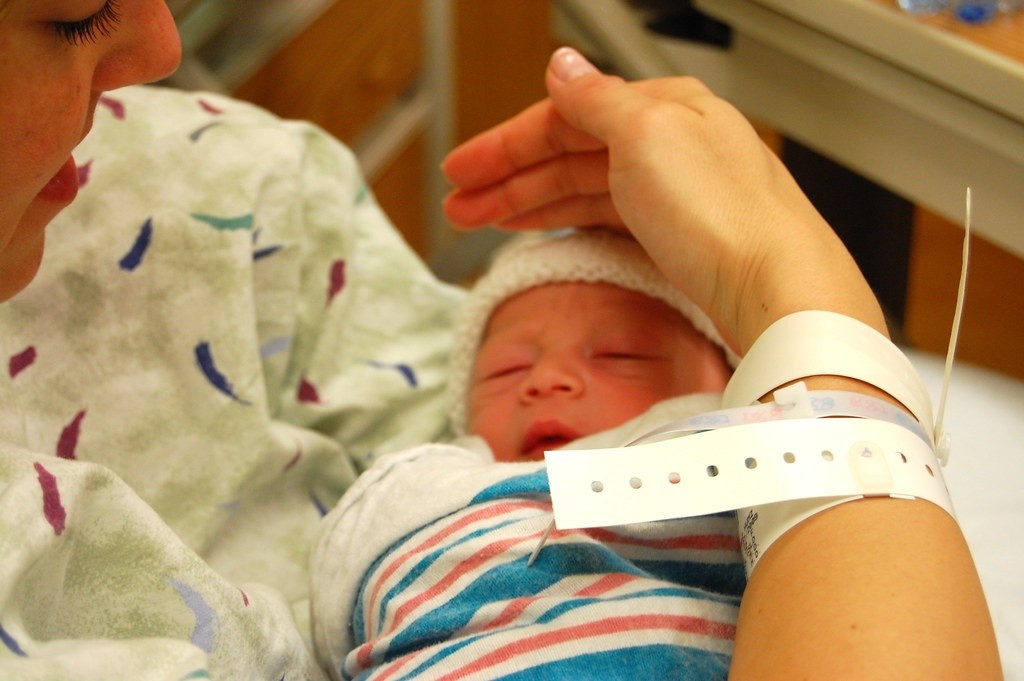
xmin=445 ymin=226 xmax=743 ymax=439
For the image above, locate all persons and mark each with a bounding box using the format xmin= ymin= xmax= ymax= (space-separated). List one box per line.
xmin=0 ymin=0 xmax=1002 ymax=681
xmin=310 ymin=230 xmax=747 ymax=681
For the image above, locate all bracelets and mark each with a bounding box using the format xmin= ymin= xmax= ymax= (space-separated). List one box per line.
xmin=544 ymin=185 xmax=973 ymax=583
